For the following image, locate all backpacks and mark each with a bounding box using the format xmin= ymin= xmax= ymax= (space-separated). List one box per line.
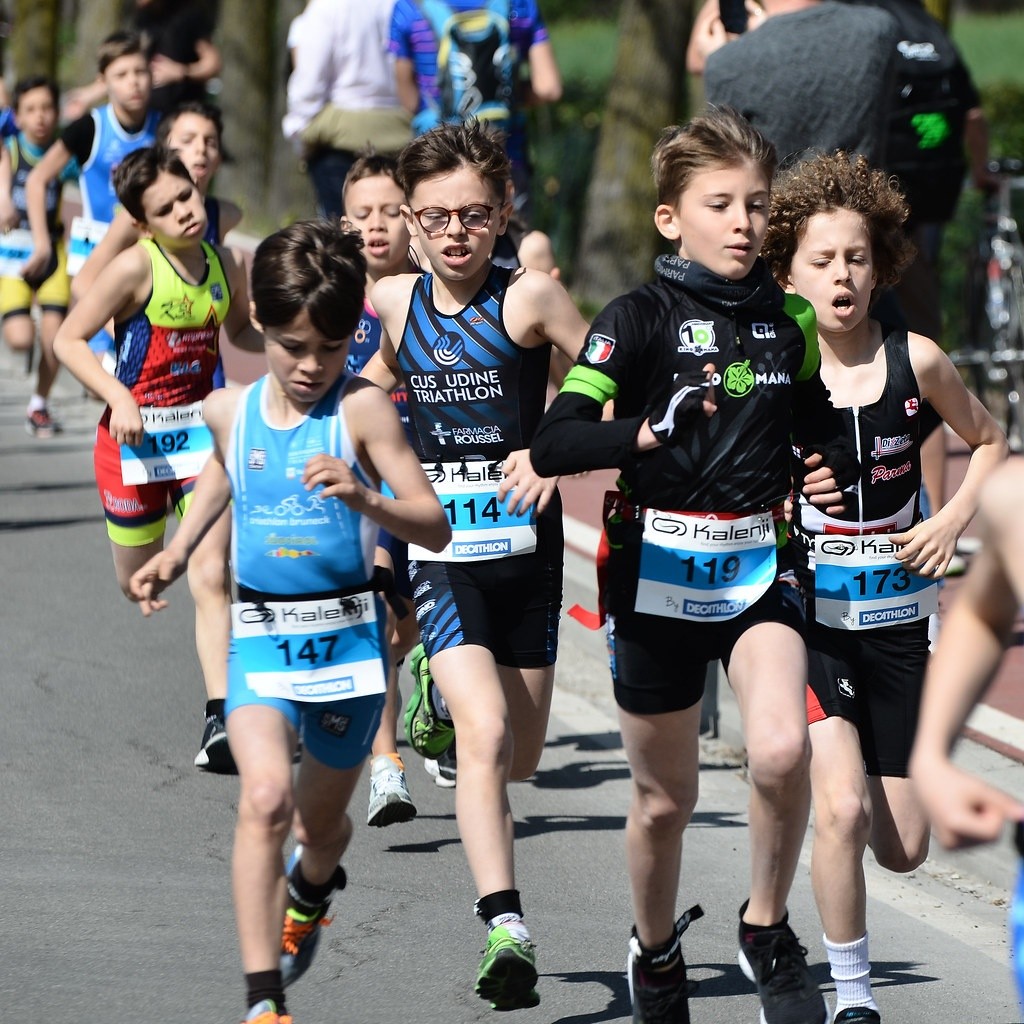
xmin=424 ymin=1 xmax=514 ymax=134
xmin=880 ymin=0 xmax=970 ymax=222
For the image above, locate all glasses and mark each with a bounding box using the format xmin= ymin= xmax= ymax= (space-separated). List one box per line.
xmin=409 ymin=202 xmax=509 ymax=233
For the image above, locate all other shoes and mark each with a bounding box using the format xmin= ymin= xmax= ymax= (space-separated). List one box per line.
xmin=833 ymin=1006 xmax=881 ymax=1024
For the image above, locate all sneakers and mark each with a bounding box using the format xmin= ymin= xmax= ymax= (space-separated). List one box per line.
xmin=277 ymin=844 xmax=344 ymax=990
xmin=195 ymin=710 xmax=237 ymax=774
xmin=631 ymin=905 xmax=704 ymax=1024
xmin=367 ymin=753 xmax=417 ymax=827
xmin=238 ymin=998 xmax=291 ymax=1024
xmin=425 ymin=749 xmax=456 ymax=788
xmin=25 ymin=409 xmax=57 ymax=439
xmin=404 ymin=643 xmax=456 ymax=760
xmin=477 ymin=922 xmax=539 ymax=1011
xmin=738 ymin=923 xmax=829 ymax=1024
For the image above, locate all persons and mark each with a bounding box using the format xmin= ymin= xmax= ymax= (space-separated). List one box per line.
xmin=529 ymin=103 xmax=865 ymax=1024
xmin=747 ymin=146 xmax=1010 ymax=1024
xmin=684 ymin=0 xmax=994 ymax=579
xmin=908 ymin=455 xmax=1024 ymax=1024
xmin=280 ymin=0 xmax=416 ymax=223
xmin=343 ymin=122 xmax=615 ymax=1011
xmin=384 ymin=0 xmax=564 ymax=259
xmin=126 ymin=217 xmax=453 ymax=1024
xmin=51 ymin=147 xmax=267 ymax=776
xmin=0 ymin=0 xmax=241 ymax=437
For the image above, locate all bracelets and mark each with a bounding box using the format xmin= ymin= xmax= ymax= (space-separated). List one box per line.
xmin=183 ymin=63 xmax=190 ymax=81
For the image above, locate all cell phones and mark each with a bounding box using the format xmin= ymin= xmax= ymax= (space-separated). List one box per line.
xmin=719 ymin=0 xmax=749 ymax=34
